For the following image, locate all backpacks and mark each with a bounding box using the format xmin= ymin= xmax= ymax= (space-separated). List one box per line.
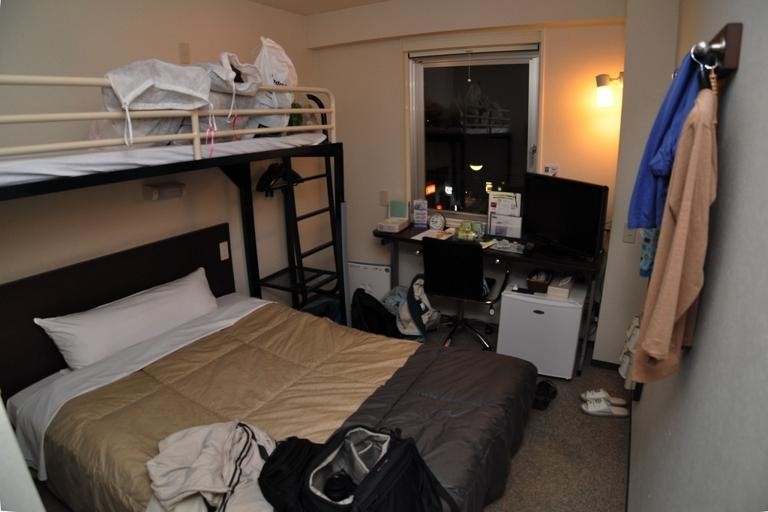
xmin=257 ymin=423 xmax=459 ymax=511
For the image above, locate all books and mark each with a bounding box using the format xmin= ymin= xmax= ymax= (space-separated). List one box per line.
xmin=487 ymin=191 xmax=522 ymax=240
xmin=411 ymin=229 xmax=453 ymax=240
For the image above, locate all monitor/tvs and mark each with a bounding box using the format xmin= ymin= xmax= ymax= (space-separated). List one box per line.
xmin=520 ymin=172 xmax=609 ymax=258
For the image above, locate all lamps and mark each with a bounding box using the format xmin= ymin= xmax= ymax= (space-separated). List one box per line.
xmin=595 ymin=71 xmax=623 ymax=107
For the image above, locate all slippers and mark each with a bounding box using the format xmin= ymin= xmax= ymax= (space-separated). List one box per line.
xmin=581 ymin=398 xmax=630 ymax=418
xmin=580 ymin=388 xmax=627 ymax=407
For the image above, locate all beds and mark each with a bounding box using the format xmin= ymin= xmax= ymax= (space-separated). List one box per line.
xmin=426 ymin=103 xmax=516 ymax=188
xmin=0 ymin=221 xmax=540 ymax=512
xmin=1 ymin=73 xmax=351 ymax=325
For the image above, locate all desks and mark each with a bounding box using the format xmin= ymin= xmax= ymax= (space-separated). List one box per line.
xmin=371 ymin=223 xmax=602 ymax=382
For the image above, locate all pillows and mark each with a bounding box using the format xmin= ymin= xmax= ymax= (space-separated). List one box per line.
xmin=32 ymin=267 xmax=218 ymax=372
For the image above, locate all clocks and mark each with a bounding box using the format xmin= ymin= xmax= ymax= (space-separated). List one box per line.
xmin=428 ymin=212 xmax=446 ymax=231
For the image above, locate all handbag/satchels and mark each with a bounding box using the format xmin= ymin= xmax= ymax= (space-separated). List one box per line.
xmin=102 ymin=53 xmax=261 ymax=150
xmin=351 ymin=279 xmax=441 ymax=340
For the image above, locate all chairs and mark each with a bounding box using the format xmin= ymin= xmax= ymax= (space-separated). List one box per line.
xmin=420 ymin=236 xmax=510 ymax=351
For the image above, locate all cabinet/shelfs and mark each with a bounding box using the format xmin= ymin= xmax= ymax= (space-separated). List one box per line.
xmin=495 ymin=275 xmax=590 ymax=379
